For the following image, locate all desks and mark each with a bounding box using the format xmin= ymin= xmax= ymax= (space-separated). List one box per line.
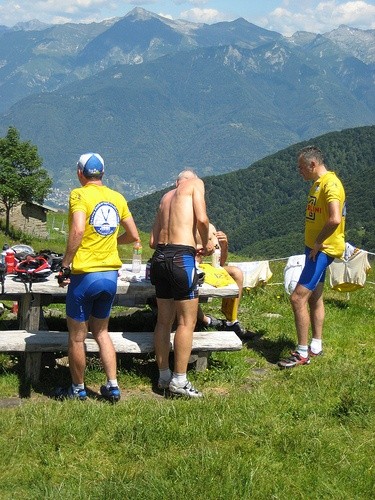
xmin=2 ymin=264 xmax=239 ymax=372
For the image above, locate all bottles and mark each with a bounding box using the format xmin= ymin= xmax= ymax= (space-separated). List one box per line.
xmin=146 ymin=258 xmax=151 ymax=280
xmin=5 ymin=252 xmax=14 ymax=274
xmin=212 ymin=246 xmax=222 ymax=267
xmin=132 ymin=240 xmax=143 ymax=273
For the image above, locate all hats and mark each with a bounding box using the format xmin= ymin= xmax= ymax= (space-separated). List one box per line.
xmin=76 ymin=151 xmax=104 ymax=178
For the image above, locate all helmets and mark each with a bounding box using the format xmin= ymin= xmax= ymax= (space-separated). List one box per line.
xmin=16 ymin=256 xmax=50 ymax=283
xmin=42 ymin=250 xmax=64 ymax=271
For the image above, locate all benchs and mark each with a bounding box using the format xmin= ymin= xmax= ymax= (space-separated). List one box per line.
xmin=0 ymin=329 xmax=242 ymax=384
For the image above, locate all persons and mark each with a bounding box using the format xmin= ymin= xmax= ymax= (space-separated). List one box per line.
xmin=57 ymin=152 xmax=140 ymax=404
xmin=150 ymin=170 xmax=210 ymax=397
xmin=198 ymin=218 xmax=243 ymax=332
xmin=276 ymin=146 xmax=347 ymax=367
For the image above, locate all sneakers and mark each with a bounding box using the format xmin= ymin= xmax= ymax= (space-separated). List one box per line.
xmin=215 ymin=320 xmax=254 ymax=340
xmin=199 ymin=315 xmax=225 ymax=327
xmin=158 ymin=379 xmax=203 ymax=398
xmin=100 ymin=386 xmax=120 ymax=403
xmin=277 ymin=347 xmax=321 ymax=367
xmin=56 ymin=386 xmax=88 ymax=400
